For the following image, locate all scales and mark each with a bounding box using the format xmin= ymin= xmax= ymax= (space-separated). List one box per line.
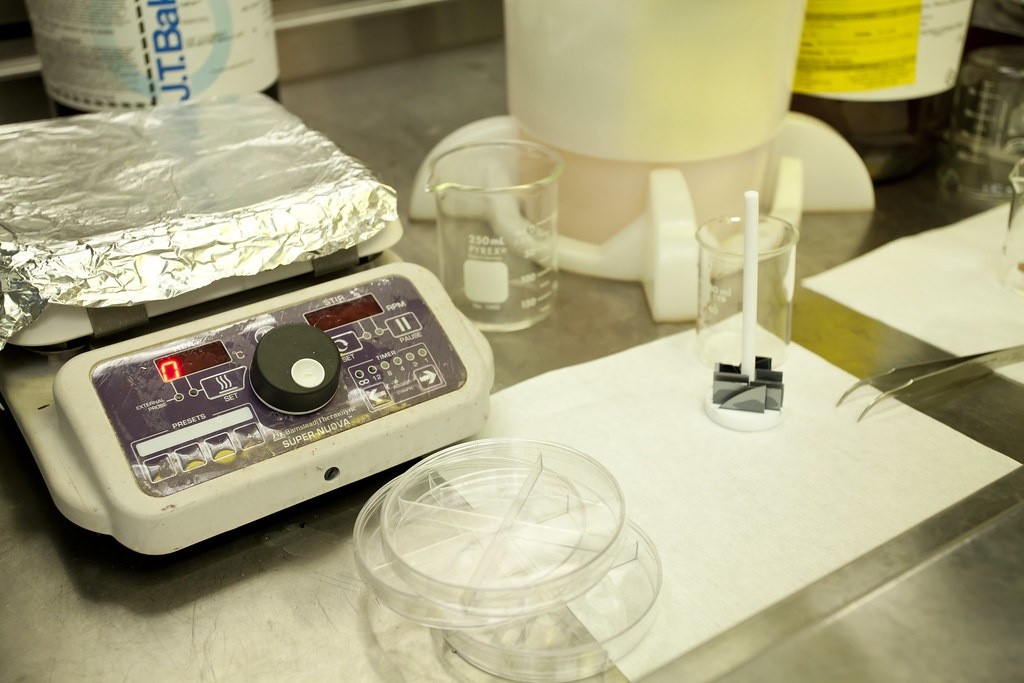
xmin=0 ymin=83 xmax=497 ymax=558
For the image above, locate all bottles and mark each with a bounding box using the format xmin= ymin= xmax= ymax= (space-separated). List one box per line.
xmin=25 ymin=0 xmax=283 ymax=119
xmin=794 ymin=0 xmax=974 ymax=187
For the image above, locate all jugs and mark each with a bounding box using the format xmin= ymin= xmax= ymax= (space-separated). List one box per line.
xmin=1002 ymin=160 xmax=1024 ymax=296
xmin=426 ymin=140 xmax=566 ymax=334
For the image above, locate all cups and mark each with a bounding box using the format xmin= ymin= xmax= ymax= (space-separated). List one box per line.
xmin=700 ymin=219 xmax=800 ymax=434
xmin=506 ymin=0 xmax=808 ymax=246
